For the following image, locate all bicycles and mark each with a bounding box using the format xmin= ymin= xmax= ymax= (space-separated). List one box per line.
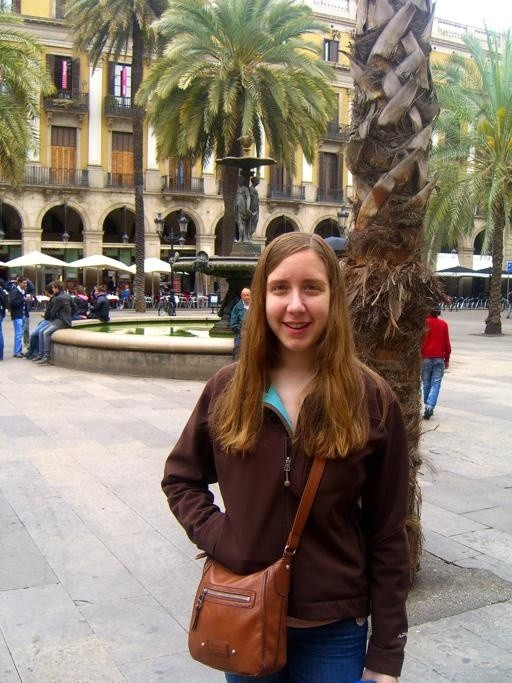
xmin=156 ymin=299 xmax=175 ymax=316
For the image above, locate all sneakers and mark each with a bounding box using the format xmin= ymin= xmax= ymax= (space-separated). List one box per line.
xmin=422 ymin=408 xmax=433 ymax=420
xmin=14 ymin=351 xmax=52 ymax=364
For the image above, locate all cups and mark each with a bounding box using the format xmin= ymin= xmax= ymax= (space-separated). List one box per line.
xmin=25 ymin=294 xmax=31 ymax=301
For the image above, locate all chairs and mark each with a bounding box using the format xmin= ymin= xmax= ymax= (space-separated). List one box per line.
xmin=34 ymin=292 xmax=208 ymax=311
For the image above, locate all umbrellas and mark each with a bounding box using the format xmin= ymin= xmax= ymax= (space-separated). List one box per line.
xmin=70 ymin=254 xmax=132 ymax=286
xmin=0 ymin=261 xmax=8 ymax=267
xmin=6 ymin=251 xmax=70 ymax=295
xmin=432 ymin=265 xmax=475 ymax=298
xmin=129 ymin=258 xmax=189 ymax=298
xmin=475 ymin=267 xmax=512 ymax=296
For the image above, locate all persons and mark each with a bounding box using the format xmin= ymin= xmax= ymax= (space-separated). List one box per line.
xmin=161 ymin=231 xmax=410 ymax=683
xmin=229 ymin=286 xmax=251 ymax=363
xmin=421 ymin=308 xmax=451 ymax=420
xmin=236 ymin=176 xmax=251 ymax=244
xmin=248 ymin=176 xmax=259 ymax=238
xmin=0 ymin=274 xmax=130 ymax=364
xmin=162 ymin=284 xmax=170 ymax=296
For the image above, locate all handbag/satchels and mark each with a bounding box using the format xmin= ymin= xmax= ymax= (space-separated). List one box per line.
xmin=188 ymin=557 xmax=293 ymax=679
